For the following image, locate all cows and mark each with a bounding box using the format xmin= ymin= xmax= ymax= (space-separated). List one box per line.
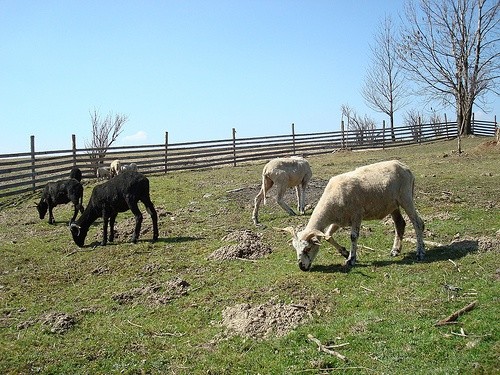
xmin=252 ymin=156 xmax=312 ymax=225
xmin=272 ymin=160 xmax=425 ymax=271
xmin=109 ymin=160 xmax=121 ymax=177
xmin=70 ymin=171 xmax=159 ymax=248
xmin=97 ymin=167 xmax=111 ymax=182
xmin=70 ymin=167 xmax=82 ymax=182
xmin=121 ymin=164 xmax=128 ymax=173
xmin=32 ymin=178 xmax=84 ymax=225
xmin=129 ymin=162 xmax=137 ymax=171
